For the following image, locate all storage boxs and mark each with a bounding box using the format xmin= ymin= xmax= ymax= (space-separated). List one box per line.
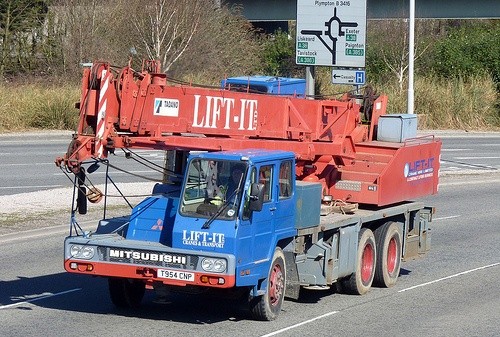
xmin=377 ymin=114 xmax=418 ymax=142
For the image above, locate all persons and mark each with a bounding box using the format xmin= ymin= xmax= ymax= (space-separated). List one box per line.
xmin=226 ymin=168 xmax=246 ymax=209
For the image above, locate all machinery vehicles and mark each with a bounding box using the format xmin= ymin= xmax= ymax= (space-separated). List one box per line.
xmin=55 ymin=56 xmax=444 ymax=322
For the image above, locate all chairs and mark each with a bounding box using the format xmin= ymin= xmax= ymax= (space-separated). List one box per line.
xmin=196 ymin=164 xmax=246 ymax=216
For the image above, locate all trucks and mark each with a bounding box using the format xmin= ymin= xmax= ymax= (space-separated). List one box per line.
xmin=220 ymin=73 xmax=306 ymax=98
